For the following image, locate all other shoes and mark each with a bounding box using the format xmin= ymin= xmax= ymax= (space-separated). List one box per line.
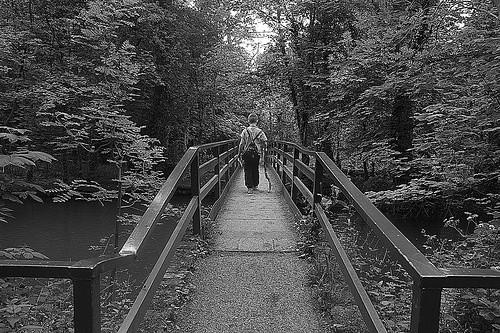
xmin=247 ymin=185 xmax=257 ymax=194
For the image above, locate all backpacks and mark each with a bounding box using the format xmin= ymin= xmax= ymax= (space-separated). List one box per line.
xmin=241 ymin=127 xmax=262 ymax=162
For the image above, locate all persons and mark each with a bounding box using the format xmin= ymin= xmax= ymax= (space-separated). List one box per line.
xmin=237 ymin=112 xmax=268 ymax=194
